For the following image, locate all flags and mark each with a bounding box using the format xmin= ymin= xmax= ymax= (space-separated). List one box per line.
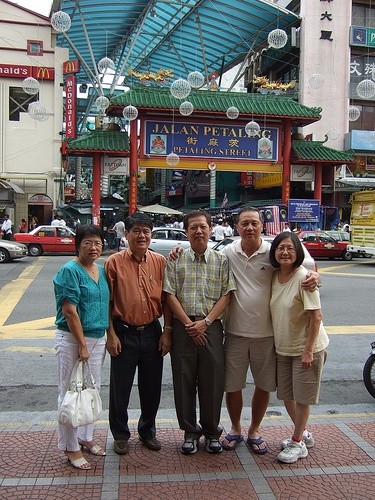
xmin=279 ymin=207 xmax=289 ymax=233
xmin=265 ymin=206 xmax=280 ymax=235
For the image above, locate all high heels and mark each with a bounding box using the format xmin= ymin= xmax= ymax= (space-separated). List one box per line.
xmin=77 ymin=437 xmax=106 ymax=455
xmin=64 ymin=450 xmax=91 ymax=469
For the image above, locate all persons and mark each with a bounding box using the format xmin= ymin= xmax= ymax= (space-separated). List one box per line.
xmin=270 ymin=231 xmax=330 ymax=463
xmin=163 ymin=211 xmax=236 ymax=453
xmin=111 ymin=218 xmax=126 ymax=252
xmin=283 ymin=223 xmax=301 ymax=233
xmin=20 ymin=218 xmax=27 ymax=233
xmin=336 ymin=222 xmax=350 ymax=233
xmin=1 ymin=214 xmax=12 ymax=240
xmin=157 ymin=215 xmax=184 ymax=229
xmin=33 ymin=218 xmax=38 ymax=228
xmin=169 ymin=208 xmax=321 ymax=454
xmin=51 ymin=215 xmax=80 ymax=236
xmin=53 ymin=224 xmax=109 ymax=469
xmin=104 ymin=212 xmax=172 ymax=453
xmin=210 ymin=221 xmax=233 ymax=242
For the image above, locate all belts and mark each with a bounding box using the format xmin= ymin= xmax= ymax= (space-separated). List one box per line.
xmin=173 ymin=316 xmax=220 ymax=323
xmin=117 ymin=318 xmax=158 ymax=331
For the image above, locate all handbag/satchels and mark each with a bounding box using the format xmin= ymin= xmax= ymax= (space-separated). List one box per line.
xmin=58 ymin=388 xmax=102 ymax=428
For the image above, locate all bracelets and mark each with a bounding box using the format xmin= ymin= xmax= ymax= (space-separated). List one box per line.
xmin=163 ymin=326 xmax=171 ymax=329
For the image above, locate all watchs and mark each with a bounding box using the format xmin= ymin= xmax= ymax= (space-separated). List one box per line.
xmin=205 ymin=318 xmax=212 ymax=325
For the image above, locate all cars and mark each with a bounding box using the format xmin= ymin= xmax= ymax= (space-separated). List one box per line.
xmin=294 ymin=230 xmax=352 ymax=260
xmin=0 ymin=239 xmax=29 ymax=263
xmin=119 ymin=227 xmax=224 ymax=261
xmin=13 ymin=226 xmax=107 ymax=257
xmin=209 ymin=236 xmax=321 ymax=288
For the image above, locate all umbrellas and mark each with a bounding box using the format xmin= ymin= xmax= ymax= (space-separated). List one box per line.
xmin=139 ymin=203 xmax=183 ymax=214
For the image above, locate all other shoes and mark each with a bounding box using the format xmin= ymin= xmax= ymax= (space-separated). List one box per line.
xmin=139 ymin=433 xmax=161 ymax=450
xmin=114 ymin=439 xmax=129 ymax=454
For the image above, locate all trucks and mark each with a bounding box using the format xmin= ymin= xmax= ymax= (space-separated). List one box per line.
xmin=346 ymin=188 xmax=375 ymax=256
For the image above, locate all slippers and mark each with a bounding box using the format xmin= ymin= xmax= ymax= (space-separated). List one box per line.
xmin=221 ymin=433 xmax=243 ymax=450
xmin=247 ymin=437 xmax=267 ymax=453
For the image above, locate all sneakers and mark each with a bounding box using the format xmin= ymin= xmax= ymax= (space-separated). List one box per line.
xmin=282 ymin=432 xmax=314 ymax=449
xmin=181 ymin=437 xmax=199 ymax=455
xmin=206 ymin=434 xmax=223 ymax=453
xmin=277 ymin=441 xmax=308 ymax=463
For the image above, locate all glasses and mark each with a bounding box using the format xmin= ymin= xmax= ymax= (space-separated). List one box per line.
xmin=275 ymin=247 xmax=297 ymax=252
xmin=81 ymin=241 xmax=103 ymax=247
